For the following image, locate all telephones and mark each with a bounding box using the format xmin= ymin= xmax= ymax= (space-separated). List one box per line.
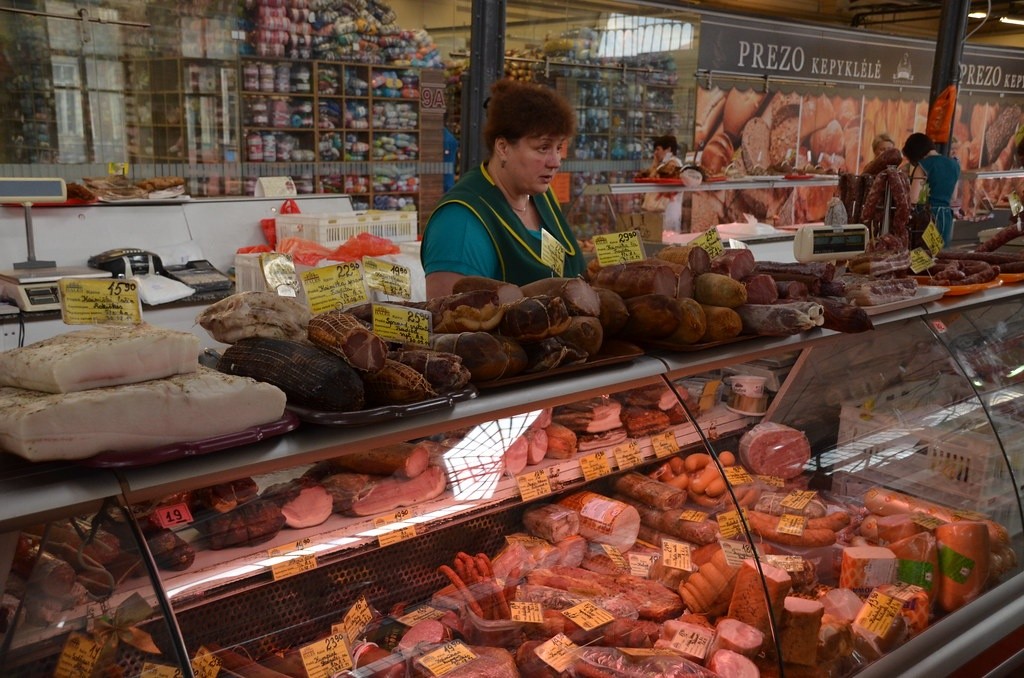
xmin=87 ymin=248 xmax=165 ymax=277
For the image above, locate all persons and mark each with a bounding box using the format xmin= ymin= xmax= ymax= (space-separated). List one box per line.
xmin=648 ymin=135 xmax=683 ymax=244
xmin=901 ymin=132 xmax=961 ymax=249
xmin=871 ymin=133 xmax=896 ymax=158
xmin=419 ymin=81 xmax=590 ymax=304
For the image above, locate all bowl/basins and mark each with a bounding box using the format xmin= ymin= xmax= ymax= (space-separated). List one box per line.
xmin=727 ymin=391 xmax=769 ymax=415
xmin=682 ymin=377 xmax=724 ymax=411
xmin=731 ymin=374 xmax=767 ymax=397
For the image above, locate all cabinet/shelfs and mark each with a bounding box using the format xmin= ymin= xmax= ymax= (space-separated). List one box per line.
xmin=0 ymin=286 xmax=1024 ymax=677
xmin=0 ymin=0 xmax=687 ymax=254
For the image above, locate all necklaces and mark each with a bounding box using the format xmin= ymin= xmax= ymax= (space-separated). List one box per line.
xmin=505 ymin=193 xmax=530 ymax=212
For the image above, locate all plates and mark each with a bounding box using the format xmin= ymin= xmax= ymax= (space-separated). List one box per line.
xmin=634 ymin=174 xmax=840 ymax=184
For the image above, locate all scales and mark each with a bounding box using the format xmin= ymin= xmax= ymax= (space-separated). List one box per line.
xmin=0 ymin=176 xmax=115 ymax=313
xmin=793 ymin=223 xmax=872 ymax=266
